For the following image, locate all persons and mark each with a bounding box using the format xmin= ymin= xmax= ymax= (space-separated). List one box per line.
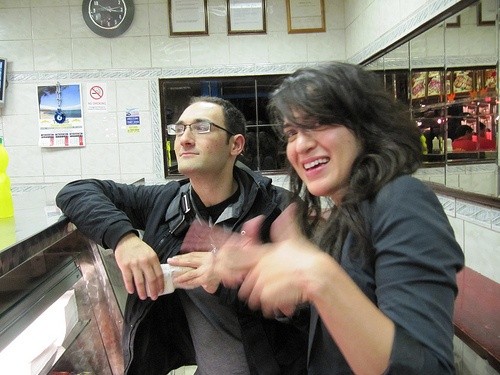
xmin=219 ymin=59 xmax=467 ymax=375
xmin=479 ymin=121 xmax=495 ymax=150
xmin=452 ymin=125 xmax=477 ymax=152
xmin=54 ymin=95 xmax=302 ymax=375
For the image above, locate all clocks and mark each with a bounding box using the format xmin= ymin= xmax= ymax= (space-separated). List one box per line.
xmin=82 ymin=0 xmax=134 ymax=38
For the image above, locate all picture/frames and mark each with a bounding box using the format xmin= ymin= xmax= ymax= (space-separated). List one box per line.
xmin=285 ymin=0 xmax=327 ymax=34
xmin=476 ymin=0 xmax=496 ymax=27
xmin=227 ymin=0 xmax=266 ymax=35
xmin=168 ymin=0 xmax=208 ymax=36
xmin=438 ymin=15 xmax=461 ymax=28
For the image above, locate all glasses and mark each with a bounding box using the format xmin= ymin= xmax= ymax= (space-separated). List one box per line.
xmin=167 ymin=120 xmax=236 ymax=136
